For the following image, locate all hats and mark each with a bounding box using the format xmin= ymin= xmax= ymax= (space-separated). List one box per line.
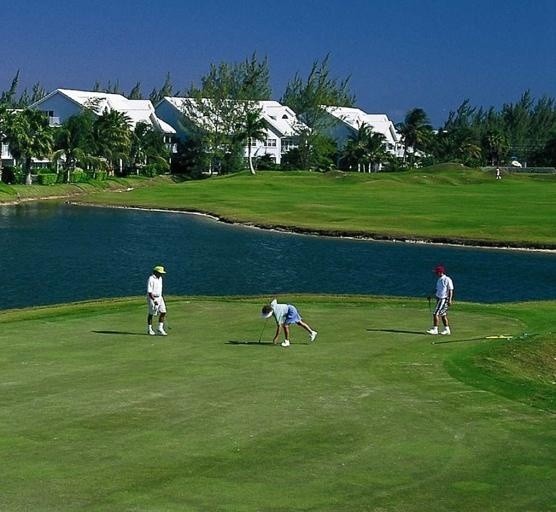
xmin=154 ymin=266 xmax=166 ymax=274
xmin=433 ymin=266 xmax=444 ymax=272
xmin=260 ymin=310 xmax=273 ymax=318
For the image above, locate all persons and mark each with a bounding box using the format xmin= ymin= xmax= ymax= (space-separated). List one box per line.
xmin=496 ymin=166 xmax=503 ymax=180
xmin=425 ymin=265 xmax=455 ymax=337
xmin=261 ymin=297 xmax=320 ymax=348
xmin=145 ymin=264 xmax=170 ymax=337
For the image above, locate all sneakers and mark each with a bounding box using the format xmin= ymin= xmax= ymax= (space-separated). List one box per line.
xmin=281 ymin=340 xmax=289 ymax=347
xmin=309 ymin=332 xmax=317 ymax=342
xmin=426 ymin=329 xmax=438 ymax=334
xmin=148 ymin=330 xmax=155 ymax=335
xmin=157 ymin=328 xmax=167 ymax=336
xmin=439 ymin=330 xmax=450 ymax=334
